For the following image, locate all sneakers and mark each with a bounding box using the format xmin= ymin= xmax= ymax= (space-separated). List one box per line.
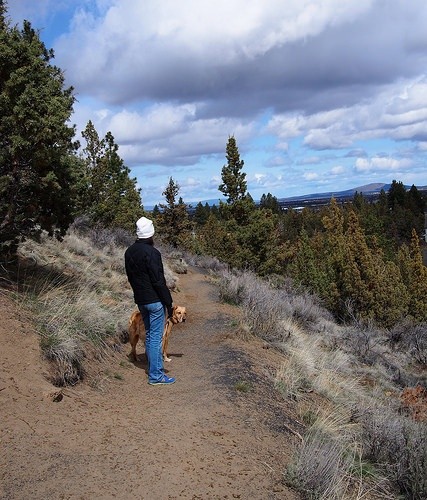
xmin=148 ymin=375 xmax=176 ymax=385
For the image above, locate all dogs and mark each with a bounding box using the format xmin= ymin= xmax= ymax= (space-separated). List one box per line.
xmin=129 ymin=305 xmax=186 ymax=363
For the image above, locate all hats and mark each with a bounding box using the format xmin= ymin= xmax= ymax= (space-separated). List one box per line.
xmin=136 ymin=217 xmax=155 ymax=239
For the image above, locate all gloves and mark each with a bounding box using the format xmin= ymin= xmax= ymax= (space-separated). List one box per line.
xmin=167 ymin=303 xmax=174 ymax=317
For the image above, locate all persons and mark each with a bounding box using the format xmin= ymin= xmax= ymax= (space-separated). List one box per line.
xmin=124 ymin=217 xmax=177 ymax=385
